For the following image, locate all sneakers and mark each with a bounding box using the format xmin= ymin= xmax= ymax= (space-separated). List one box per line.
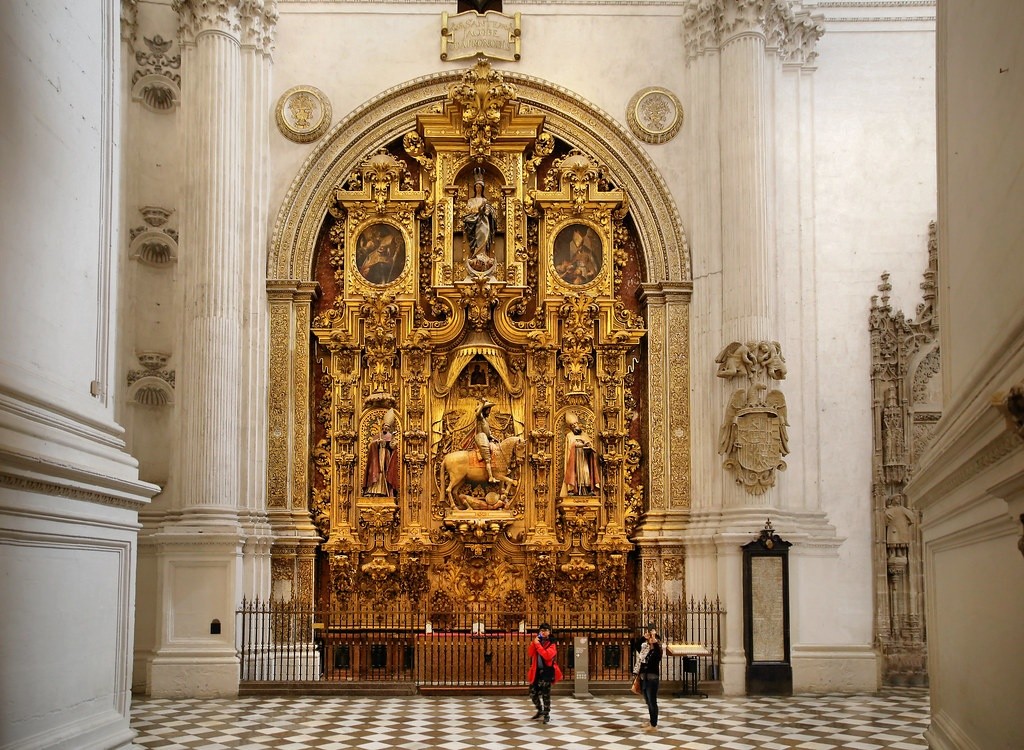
xmin=531 ymin=711 xmax=544 ymax=719
xmin=543 ymin=716 xmax=550 ymax=724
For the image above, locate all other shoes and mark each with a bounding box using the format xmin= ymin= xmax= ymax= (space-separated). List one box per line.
xmin=642 ymin=721 xmax=655 ymax=728
xmin=644 ymin=726 xmax=657 ymax=734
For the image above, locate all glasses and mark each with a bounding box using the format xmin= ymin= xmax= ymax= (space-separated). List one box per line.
xmin=540 ymin=629 xmax=549 ymax=633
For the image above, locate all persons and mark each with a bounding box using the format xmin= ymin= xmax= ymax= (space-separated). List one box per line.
xmin=565 ymin=409 xmax=597 ymax=496
xmin=528 ymin=623 xmax=557 ymax=724
xmin=365 ymin=409 xmax=396 ymax=497
xmin=758 ymin=341 xmax=787 ymax=380
xmin=463 ymin=167 xmax=494 ymax=257
xmin=717 ymin=340 xmax=757 ymax=379
xmin=474 ymin=397 xmax=501 ymax=483
xmin=634 ymin=629 xmax=663 ymax=731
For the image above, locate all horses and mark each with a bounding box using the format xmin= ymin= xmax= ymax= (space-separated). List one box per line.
xmin=439 ymin=437 xmax=529 ymax=510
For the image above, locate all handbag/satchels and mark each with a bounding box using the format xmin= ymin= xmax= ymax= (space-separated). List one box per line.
xmin=630 ymin=675 xmax=643 ymax=694
xmin=543 ymin=666 xmax=555 ymax=682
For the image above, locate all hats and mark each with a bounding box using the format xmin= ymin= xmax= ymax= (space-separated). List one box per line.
xmin=650 ymin=634 xmax=661 ymax=642
xmin=539 ymin=623 xmax=551 ymax=629
